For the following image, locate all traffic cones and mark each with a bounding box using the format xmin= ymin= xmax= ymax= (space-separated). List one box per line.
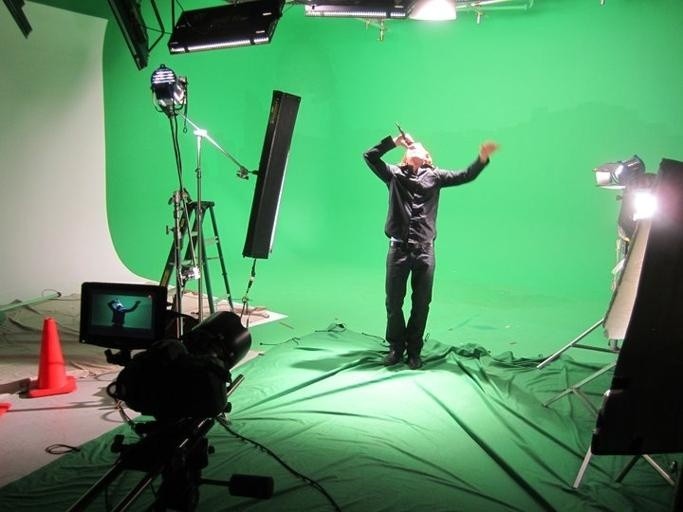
xmin=27 ymin=316 xmax=78 ymax=398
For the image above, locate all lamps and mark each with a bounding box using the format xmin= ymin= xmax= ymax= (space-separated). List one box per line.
xmin=106 ymin=0 xmax=304 ymax=261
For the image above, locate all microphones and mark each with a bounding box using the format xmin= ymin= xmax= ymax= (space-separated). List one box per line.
xmin=394 ymin=122 xmax=415 ymax=150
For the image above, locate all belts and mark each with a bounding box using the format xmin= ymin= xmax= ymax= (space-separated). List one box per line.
xmin=389 ymin=240 xmax=433 ymax=248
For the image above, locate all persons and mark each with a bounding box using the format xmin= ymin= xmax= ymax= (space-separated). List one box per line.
xmin=107 ymin=298 xmax=141 ymax=327
xmin=363 ymin=133 xmax=496 ymax=371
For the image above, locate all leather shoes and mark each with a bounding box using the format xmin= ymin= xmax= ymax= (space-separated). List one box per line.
xmin=406 ymin=344 xmax=422 ymax=369
xmin=384 ymin=346 xmax=406 ymax=366
